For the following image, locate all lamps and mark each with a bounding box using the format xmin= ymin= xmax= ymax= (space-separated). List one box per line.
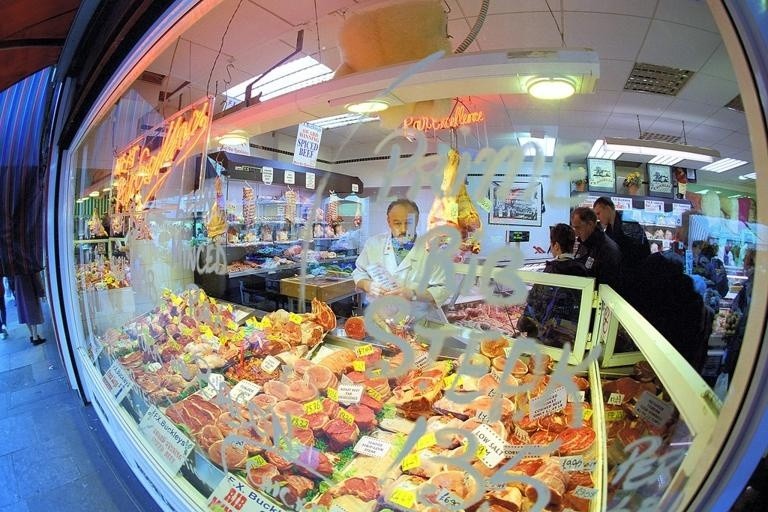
xmin=328 ymin=88 xmax=408 ymax=113
xmin=76 ymin=161 xmax=172 ymax=204
xmin=604 ymin=114 xmax=720 ymax=163
xmin=525 ymin=75 xmax=577 ymax=99
xmin=218 ymin=136 xmax=248 ymax=145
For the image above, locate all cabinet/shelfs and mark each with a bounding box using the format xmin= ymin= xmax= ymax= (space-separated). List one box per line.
xmin=193 ymin=151 xmax=364 ymax=310
xmin=568 ymin=190 xmax=756 ymax=364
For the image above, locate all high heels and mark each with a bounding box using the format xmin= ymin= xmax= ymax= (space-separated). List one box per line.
xmin=0 ymin=329 xmax=8 ymax=340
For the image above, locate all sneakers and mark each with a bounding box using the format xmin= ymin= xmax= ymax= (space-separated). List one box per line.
xmin=30 ymin=335 xmax=46 ymax=346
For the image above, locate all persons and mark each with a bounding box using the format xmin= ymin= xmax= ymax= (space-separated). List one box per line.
xmin=515 ymin=197 xmax=755 ymax=426
xmin=8 ymin=274 xmax=47 ymax=346
xmin=0 ymin=257 xmax=8 ymax=340
xmin=350 ymin=199 xmax=463 ymax=340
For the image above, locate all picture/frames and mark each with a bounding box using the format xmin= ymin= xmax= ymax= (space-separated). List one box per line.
xmin=488 ymin=181 xmax=543 ymax=227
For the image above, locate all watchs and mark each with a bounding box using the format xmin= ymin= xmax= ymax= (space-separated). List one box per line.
xmin=409 ymin=288 xmax=416 ymax=302
